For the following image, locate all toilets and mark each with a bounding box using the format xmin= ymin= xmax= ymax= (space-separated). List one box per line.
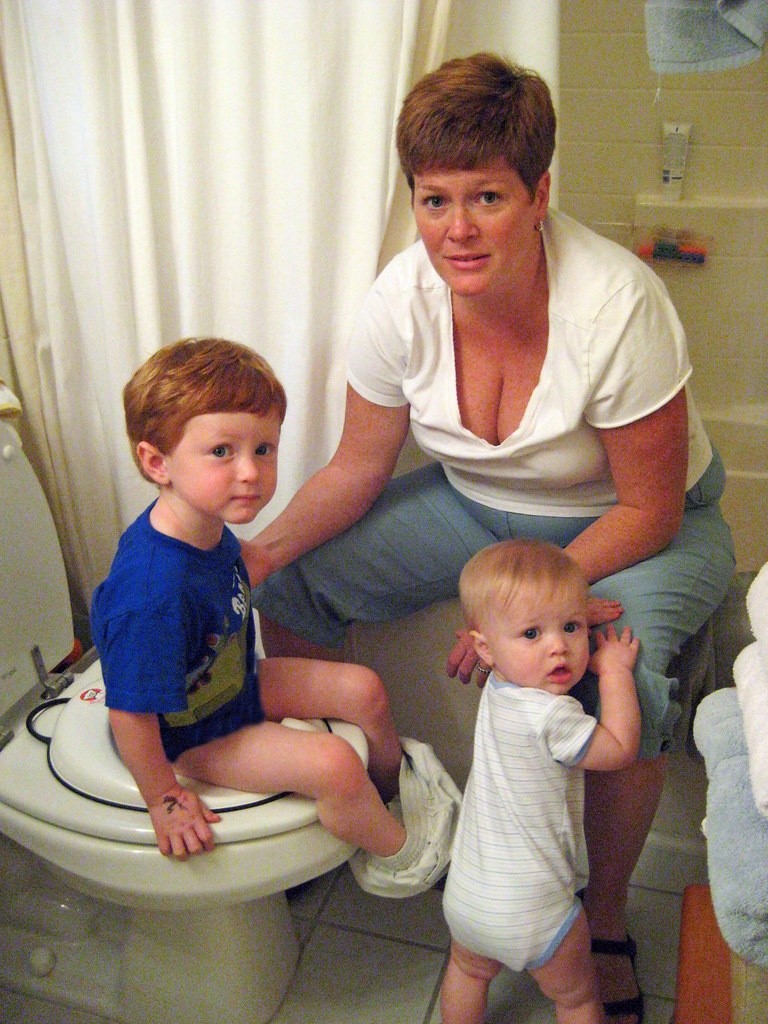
xmin=1 ymin=422 xmax=374 ymax=1024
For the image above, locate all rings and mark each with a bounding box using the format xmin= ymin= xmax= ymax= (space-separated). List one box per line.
xmin=476 ymin=664 xmax=490 ymax=675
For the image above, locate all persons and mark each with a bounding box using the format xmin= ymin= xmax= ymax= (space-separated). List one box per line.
xmin=438 ymin=536 xmax=641 ymax=1024
xmin=236 ymin=51 xmax=737 ymax=1024
xmin=87 ymin=338 xmax=464 ymax=899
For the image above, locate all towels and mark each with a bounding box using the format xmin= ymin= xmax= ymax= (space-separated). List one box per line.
xmin=690 ymin=559 xmax=768 ymax=969
xmin=644 ymin=0 xmax=768 ymax=74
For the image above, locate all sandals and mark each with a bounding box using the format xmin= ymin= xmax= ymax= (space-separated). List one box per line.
xmin=591 ymin=929 xmax=645 ymax=1024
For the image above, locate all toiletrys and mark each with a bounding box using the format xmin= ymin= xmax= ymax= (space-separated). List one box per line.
xmin=660 ymin=121 xmax=692 ymax=200
